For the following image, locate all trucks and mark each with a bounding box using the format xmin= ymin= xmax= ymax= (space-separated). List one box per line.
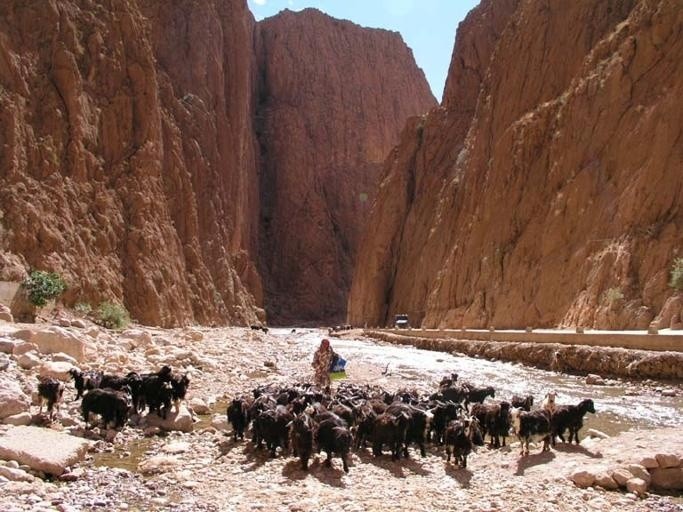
xmin=395 ymin=315 xmax=409 ymax=329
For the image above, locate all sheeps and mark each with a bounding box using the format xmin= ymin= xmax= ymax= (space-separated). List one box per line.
xmin=248 ymin=324 xmax=268 ymax=335
xmin=65 ymin=365 xmax=191 ymax=431
xmin=508 ymin=391 xmax=597 ymax=455
xmin=35 ymin=374 xmax=67 ymax=422
xmin=227 ymin=373 xmax=509 ymax=475
xmin=327 ymin=323 xmax=353 ymax=335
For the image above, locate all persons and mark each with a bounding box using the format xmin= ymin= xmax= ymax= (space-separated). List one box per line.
xmin=311 ymin=339 xmax=336 ymax=387
